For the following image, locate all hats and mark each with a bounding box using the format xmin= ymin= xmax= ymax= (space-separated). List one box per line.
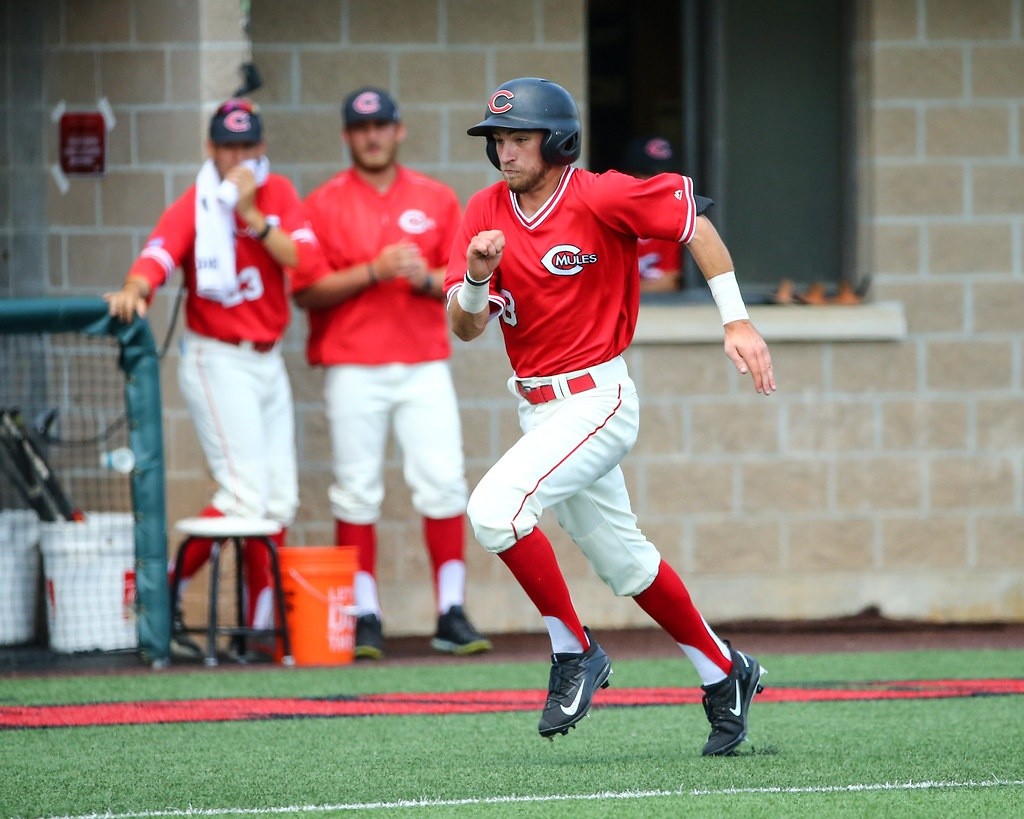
xmin=637 ymin=138 xmax=676 ymax=175
xmin=343 ymin=86 xmax=399 ymax=131
xmin=209 ymin=103 xmax=263 ymax=147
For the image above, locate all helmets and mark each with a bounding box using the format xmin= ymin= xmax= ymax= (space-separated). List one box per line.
xmin=467 ymin=77 xmax=583 ymax=171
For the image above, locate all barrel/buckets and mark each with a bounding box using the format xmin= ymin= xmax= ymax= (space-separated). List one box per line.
xmin=0 ymin=508 xmax=39 ymax=647
xmin=38 ymin=510 xmax=136 ymax=655
xmin=275 ymin=544 xmax=361 ymax=666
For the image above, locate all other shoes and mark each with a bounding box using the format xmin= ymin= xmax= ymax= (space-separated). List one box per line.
xmin=168 ymin=607 xmax=202 ymax=656
xmin=224 ymin=646 xmax=273 ymax=665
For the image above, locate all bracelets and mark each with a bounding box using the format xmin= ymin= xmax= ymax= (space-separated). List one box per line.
xmin=420 ymin=266 xmax=432 ymax=294
xmin=253 ymin=222 xmax=271 ymax=240
xmin=120 ymin=278 xmax=147 ymax=299
xmin=365 ymin=257 xmax=379 ymax=286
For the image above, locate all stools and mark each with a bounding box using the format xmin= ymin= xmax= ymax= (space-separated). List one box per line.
xmin=171 ymin=514 xmax=288 ymax=670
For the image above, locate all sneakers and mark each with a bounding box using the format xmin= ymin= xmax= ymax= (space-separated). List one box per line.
xmin=354 ymin=614 xmax=385 ymax=660
xmin=701 ymin=639 xmax=767 ymax=756
xmin=429 ymin=605 xmax=493 ymax=656
xmin=538 ymin=626 xmax=613 ymax=742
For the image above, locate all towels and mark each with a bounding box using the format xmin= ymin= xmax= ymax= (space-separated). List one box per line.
xmin=194 ymin=151 xmax=270 ymax=304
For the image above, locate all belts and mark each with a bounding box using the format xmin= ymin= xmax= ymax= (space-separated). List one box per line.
xmin=219 ymin=336 xmax=276 ymax=352
xmin=515 ymin=373 xmax=596 ymax=405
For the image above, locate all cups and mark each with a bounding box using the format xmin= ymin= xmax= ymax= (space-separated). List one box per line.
xmin=99 ymin=447 xmax=135 ymax=474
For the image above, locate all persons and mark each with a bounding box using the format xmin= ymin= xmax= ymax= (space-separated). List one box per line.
xmin=443 ymin=78 xmax=778 ymax=756
xmin=282 ymin=86 xmax=493 ymax=659
xmin=100 ymin=95 xmax=332 ymax=669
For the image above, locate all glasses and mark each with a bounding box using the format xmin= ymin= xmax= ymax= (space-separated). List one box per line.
xmin=212 ymin=99 xmax=261 ymax=124
